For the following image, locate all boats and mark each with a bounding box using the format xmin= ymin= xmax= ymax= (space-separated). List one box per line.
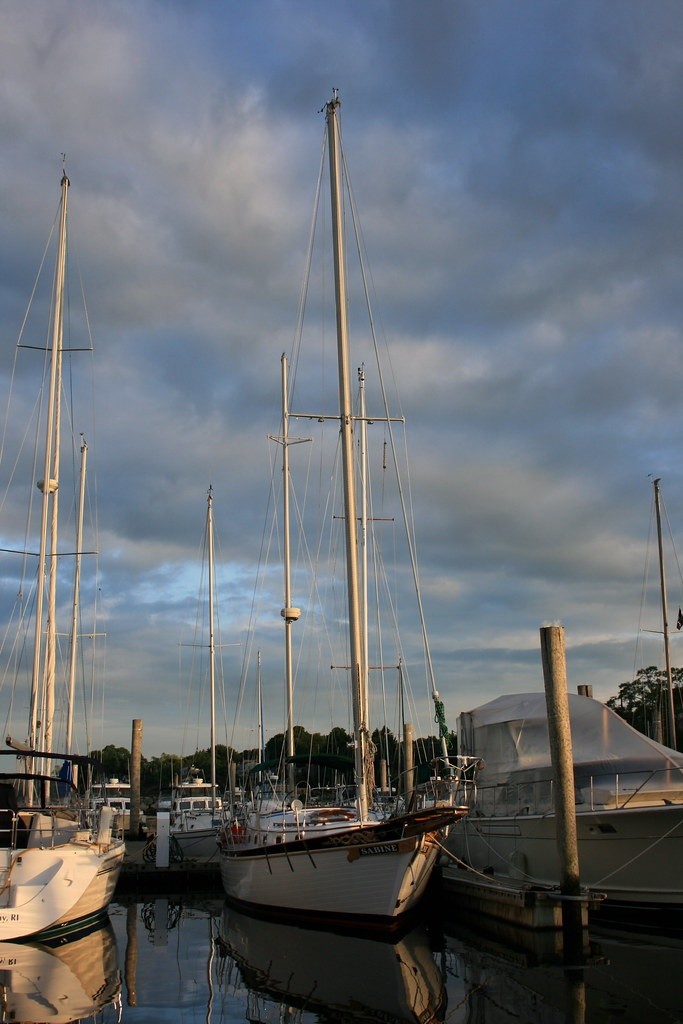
xmin=352 ymin=780 xmax=405 ymax=814
xmin=440 ymin=866 xmax=589 ymax=930
xmin=436 ymin=690 xmax=683 ymax=919
xmin=167 ymin=765 xmax=231 ymax=861
xmin=415 ymin=775 xmax=456 ymax=810
xmin=87 ymin=780 xmax=149 ymax=841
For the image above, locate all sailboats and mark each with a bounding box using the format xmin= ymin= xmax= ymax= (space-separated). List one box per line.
xmin=217 ymin=907 xmax=449 ymax=1024
xmin=219 ymin=645 xmax=287 ymax=817
xmin=0 ymin=174 xmax=124 ymax=951
xmin=217 ymin=95 xmax=470 ymax=938
xmin=0 ymin=916 xmax=119 ymax=1024
